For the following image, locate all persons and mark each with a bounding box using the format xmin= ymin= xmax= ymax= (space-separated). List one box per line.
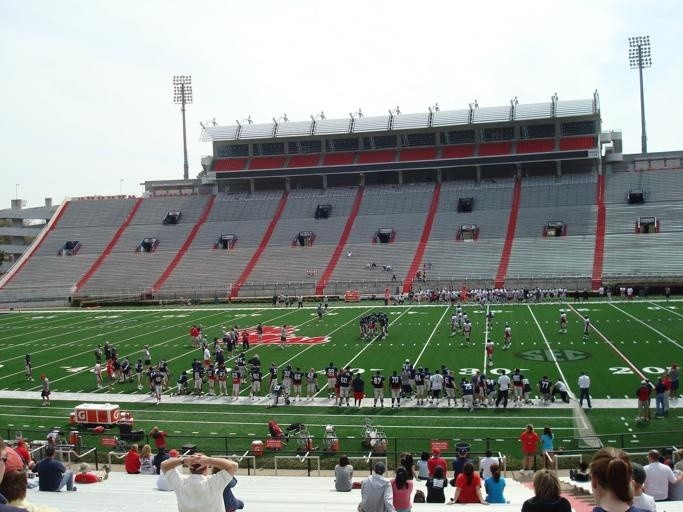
xmin=516 ymin=425 xmax=681 ymax=512
xmin=0 ymin=423 xmax=111 ymax=511
xmin=271 ymin=293 xmax=329 ymax=319
xmin=342 ymin=251 xmax=431 ymax=343
xmin=116 ymin=422 xmax=245 ymax=512
xmin=38 ymin=373 xmax=50 ymax=408
xmin=92 ymin=339 xmax=171 ymax=405
xmin=429 ymin=286 xmax=633 ymax=367
xmin=23 ymin=351 xmax=32 ymax=383
xmin=174 ymin=322 xmax=264 ymax=400
xmin=278 ymin=325 xmax=288 ymax=349
xmin=267 ymin=423 xmax=507 ymax=511
xmin=267 ymin=358 xmax=592 ymax=411
xmin=636 ymin=363 xmax=680 ymax=419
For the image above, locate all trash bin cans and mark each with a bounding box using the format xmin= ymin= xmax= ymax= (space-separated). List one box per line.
xmin=455 ymin=442 xmax=471 ymax=460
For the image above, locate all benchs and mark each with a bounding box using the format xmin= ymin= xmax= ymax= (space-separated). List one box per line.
xmin=0 ymin=452 xmax=683 ymax=512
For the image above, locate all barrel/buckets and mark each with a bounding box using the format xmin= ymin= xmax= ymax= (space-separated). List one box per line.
xmin=69 ymin=412 xmax=75 ymax=425
xmin=69 ymin=431 xmax=79 ymax=446
xmin=251 ymin=440 xmax=263 ymax=456
xmin=93 ymin=425 xmax=104 ymax=434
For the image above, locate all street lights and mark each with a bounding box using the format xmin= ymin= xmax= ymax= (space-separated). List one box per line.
xmin=119 ymin=178 xmax=124 ymax=196
xmin=15 ymin=183 xmax=20 ymax=200
xmin=171 ymin=75 xmax=192 ymax=180
xmin=627 ymin=34 xmax=653 ymax=155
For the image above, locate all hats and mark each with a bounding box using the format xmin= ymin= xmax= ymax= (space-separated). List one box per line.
xmin=170 ymin=449 xmax=179 ymax=456
xmin=432 ymin=447 xmax=440 ymax=454
xmin=78 ymin=462 xmax=91 ymax=473
